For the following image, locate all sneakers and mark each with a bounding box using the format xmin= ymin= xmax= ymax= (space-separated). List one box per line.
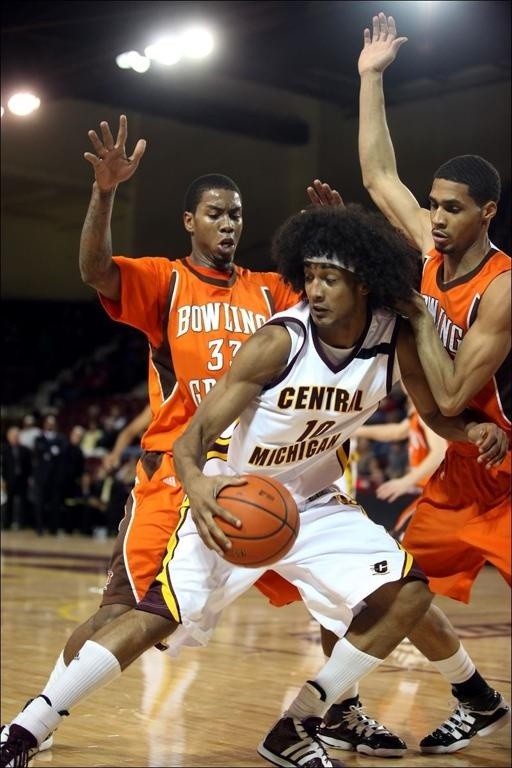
xmin=317 ymin=695 xmax=408 ymax=758
xmin=420 ymin=688 xmax=511 ymax=754
xmin=0 ymin=699 xmax=54 ymax=767
xmin=257 ymin=717 xmax=333 ymax=766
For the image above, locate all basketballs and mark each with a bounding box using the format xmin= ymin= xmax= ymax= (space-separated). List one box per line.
xmin=213 ymin=474 xmax=300 ymax=569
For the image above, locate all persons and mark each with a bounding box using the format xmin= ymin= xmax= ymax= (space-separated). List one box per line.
xmin=27 ymin=113 xmax=347 ymax=752
xmin=0 ymin=201 xmax=509 ymax=768
xmin=0 ymin=355 xmax=146 ymax=536
xmin=351 ymin=377 xmax=450 ymax=534
xmin=356 ymin=11 xmax=510 ymax=756
xmin=101 ymin=404 xmax=171 ymax=652
xmin=358 ymin=381 xmax=424 ymax=511
